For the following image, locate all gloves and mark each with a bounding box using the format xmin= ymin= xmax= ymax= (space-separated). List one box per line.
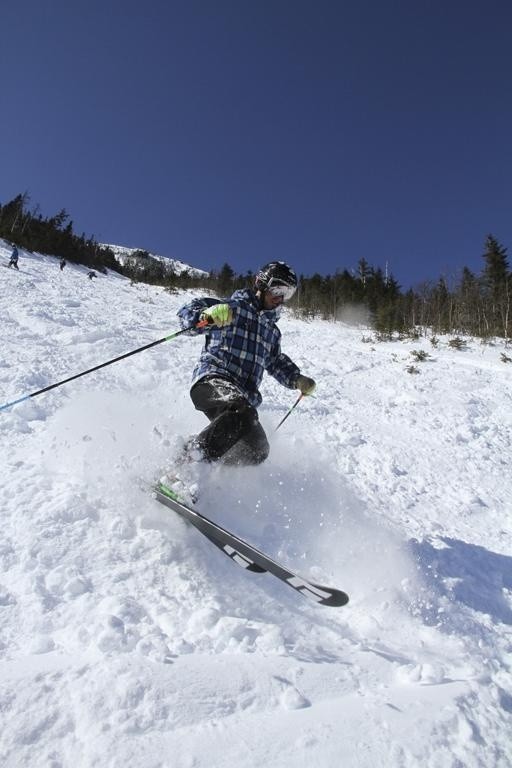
xmin=295 ymin=375 xmax=318 ymax=397
xmin=198 ymin=301 xmax=234 ymax=332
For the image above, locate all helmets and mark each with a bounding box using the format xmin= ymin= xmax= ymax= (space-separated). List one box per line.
xmin=254 ymin=260 xmax=299 ymax=309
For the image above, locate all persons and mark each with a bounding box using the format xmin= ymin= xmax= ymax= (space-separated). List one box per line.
xmin=8 ymin=242 xmax=20 ymax=272
xmin=87 ymin=270 xmax=98 ymax=282
xmin=176 ymin=256 xmax=318 ymax=493
xmin=60 ymin=258 xmax=66 ymax=272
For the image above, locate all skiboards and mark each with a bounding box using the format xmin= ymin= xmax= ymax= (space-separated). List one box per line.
xmin=141 ymin=480 xmax=349 ymax=608
